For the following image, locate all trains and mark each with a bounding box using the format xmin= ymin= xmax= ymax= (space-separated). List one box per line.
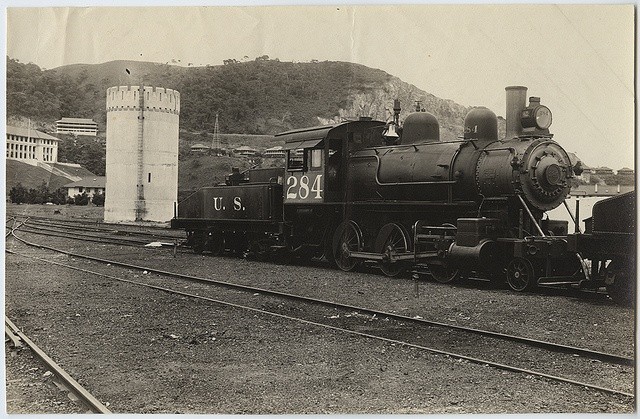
xmin=172 ymin=86 xmax=636 ymax=302
